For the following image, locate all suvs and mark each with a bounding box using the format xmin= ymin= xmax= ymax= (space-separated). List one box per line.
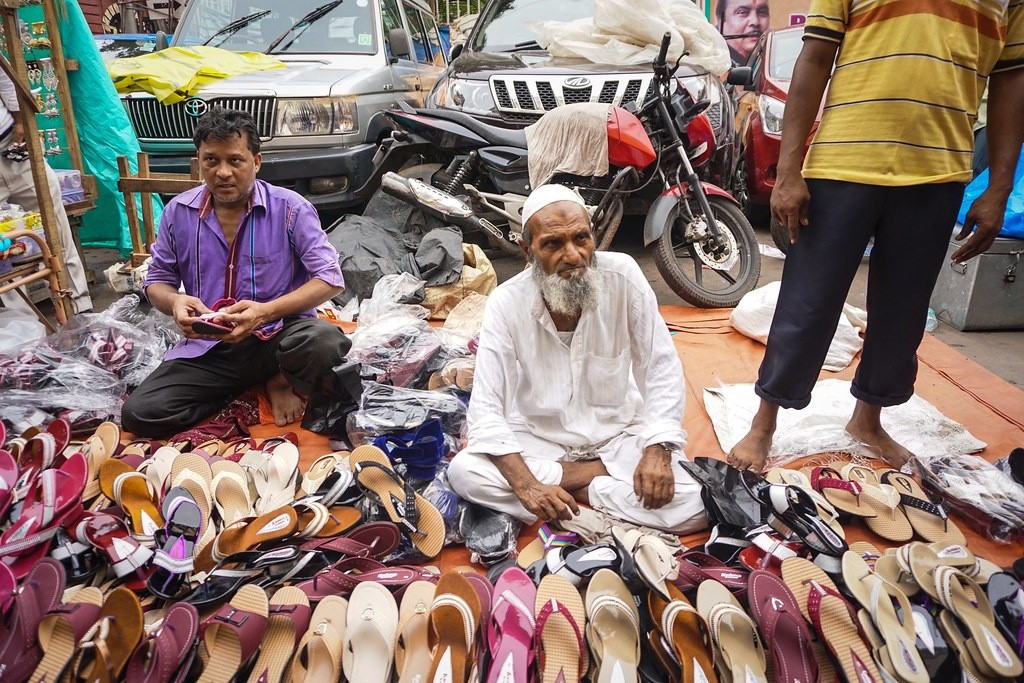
xmin=424 ymin=0 xmax=754 ymax=220
xmin=116 ymin=0 xmax=449 ymax=210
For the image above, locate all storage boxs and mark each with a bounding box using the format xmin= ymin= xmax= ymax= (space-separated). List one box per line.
xmin=929 ymin=221 xmax=1024 ymax=333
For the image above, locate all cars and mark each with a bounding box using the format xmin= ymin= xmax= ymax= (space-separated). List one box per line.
xmin=730 ymin=25 xmax=839 ymax=227
xmin=93 ymin=35 xmax=203 ymax=63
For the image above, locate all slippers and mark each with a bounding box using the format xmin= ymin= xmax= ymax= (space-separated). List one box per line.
xmin=191 ymin=299 xmax=238 ymax=334
xmin=0 ymin=418 xmax=1023 ymax=683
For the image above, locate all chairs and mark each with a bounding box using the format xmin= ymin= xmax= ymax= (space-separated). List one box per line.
xmin=251 ymin=12 xmax=376 ymax=54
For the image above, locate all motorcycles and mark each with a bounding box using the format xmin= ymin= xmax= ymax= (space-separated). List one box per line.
xmin=353 ymin=31 xmax=761 ymax=309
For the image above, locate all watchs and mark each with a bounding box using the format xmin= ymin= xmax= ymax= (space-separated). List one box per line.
xmin=660 ymin=442 xmax=675 ymax=452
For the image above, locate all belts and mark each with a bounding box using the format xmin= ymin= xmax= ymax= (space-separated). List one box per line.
xmin=0 ymin=121 xmax=15 ymax=141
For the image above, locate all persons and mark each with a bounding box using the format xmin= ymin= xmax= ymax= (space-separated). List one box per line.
xmin=713 ymin=0 xmax=771 ymax=95
xmin=727 ymin=0 xmax=1024 ymax=475
xmin=446 ymin=184 xmax=709 ymax=536
xmin=120 ymin=107 xmax=352 ymax=436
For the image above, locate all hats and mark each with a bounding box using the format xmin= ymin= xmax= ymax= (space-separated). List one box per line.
xmin=521 ymin=183 xmax=586 ymax=232
